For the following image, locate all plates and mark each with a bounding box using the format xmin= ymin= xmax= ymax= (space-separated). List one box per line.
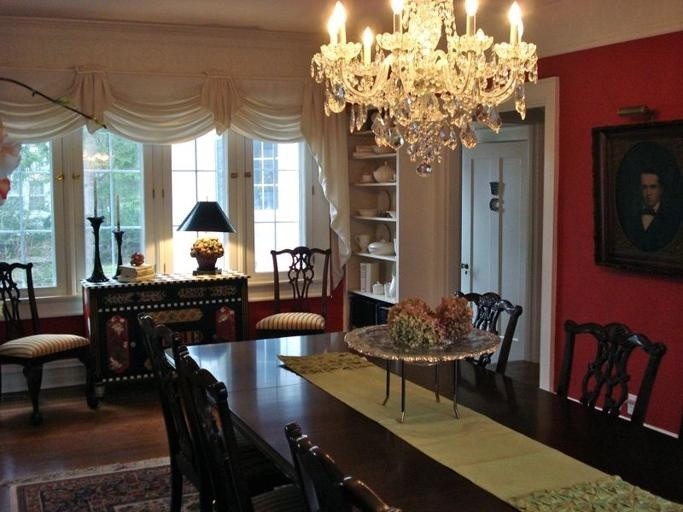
xmin=377 ymin=190 xmax=390 ymax=211
xmin=352 ymin=143 xmax=397 ymax=157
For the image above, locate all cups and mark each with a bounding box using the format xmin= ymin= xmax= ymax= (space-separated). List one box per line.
xmin=355 ymin=224 xmax=396 ymax=257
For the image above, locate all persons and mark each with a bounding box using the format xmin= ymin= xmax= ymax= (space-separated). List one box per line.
xmin=623 ymin=170 xmax=676 ymax=252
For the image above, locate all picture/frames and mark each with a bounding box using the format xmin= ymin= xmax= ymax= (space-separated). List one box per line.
xmin=590 ymin=118 xmax=683 ymax=279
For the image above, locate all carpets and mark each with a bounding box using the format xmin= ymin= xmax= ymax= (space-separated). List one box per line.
xmin=0 ymin=457 xmax=201 ymax=512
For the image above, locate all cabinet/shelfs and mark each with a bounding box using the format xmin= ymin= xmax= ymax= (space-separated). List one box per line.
xmin=344 ymin=103 xmax=462 ymax=331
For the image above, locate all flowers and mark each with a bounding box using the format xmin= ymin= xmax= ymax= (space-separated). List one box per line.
xmin=190 ymin=236 xmax=225 ymax=258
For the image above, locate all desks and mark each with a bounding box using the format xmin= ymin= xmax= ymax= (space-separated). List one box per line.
xmin=78 ymin=269 xmax=250 ymax=409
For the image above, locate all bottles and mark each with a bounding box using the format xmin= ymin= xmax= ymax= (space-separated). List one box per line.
xmin=360 ymin=260 xmax=396 ymax=299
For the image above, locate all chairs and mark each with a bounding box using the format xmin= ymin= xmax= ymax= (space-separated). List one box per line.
xmin=253 ymin=246 xmax=331 ymax=340
xmin=0 ymin=260 xmax=96 ymax=427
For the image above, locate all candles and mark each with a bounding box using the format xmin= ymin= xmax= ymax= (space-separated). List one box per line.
xmin=117 ymin=195 xmax=120 ymax=232
xmin=94 ymin=175 xmax=97 ymax=217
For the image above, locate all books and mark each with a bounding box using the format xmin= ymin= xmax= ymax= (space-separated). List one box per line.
xmin=117 ymin=274 xmax=155 ymax=283
xmin=121 ymin=269 xmax=153 ymax=277
xmin=117 ymin=265 xmax=152 ymax=272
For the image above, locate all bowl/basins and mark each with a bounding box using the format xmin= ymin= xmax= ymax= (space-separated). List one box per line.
xmin=355 ymin=208 xmax=396 ymax=218
xmin=359 ymin=160 xmax=398 ymax=183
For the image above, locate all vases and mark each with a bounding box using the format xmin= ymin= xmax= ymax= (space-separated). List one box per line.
xmin=196 ymin=258 xmax=217 ymax=269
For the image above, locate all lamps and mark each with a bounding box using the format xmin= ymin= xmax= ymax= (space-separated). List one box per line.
xmin=306 ymin=1 xmax=544 ymax=179
xmin=175 ymin=200 xmax=236 ymax=234
